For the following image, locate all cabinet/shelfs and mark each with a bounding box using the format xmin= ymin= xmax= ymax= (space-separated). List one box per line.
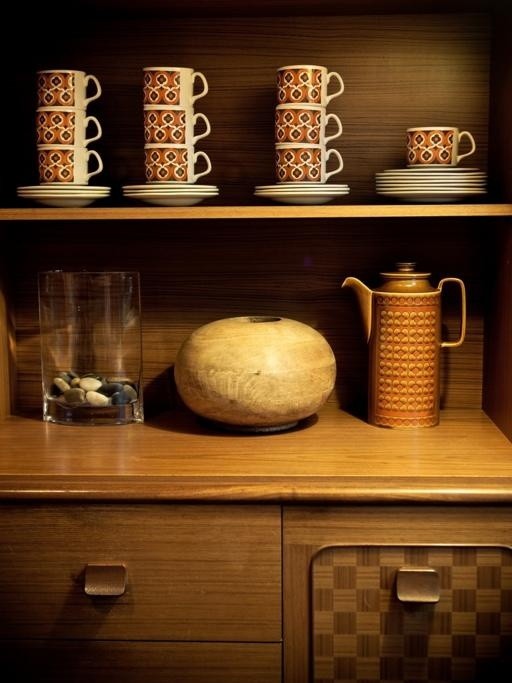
xmin=0 ymin=0 xmax=511 ymax=683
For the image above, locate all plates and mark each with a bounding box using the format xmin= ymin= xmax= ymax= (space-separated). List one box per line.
xmin=15 ymin=168 xmax=490 ymax=208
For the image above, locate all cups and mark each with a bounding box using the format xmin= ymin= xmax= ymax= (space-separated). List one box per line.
xmin=142 ymin=67 xmax=209 ymax=106
xmin=275 ymin=105 xmax=343 ymax=142
xmin=37 ymin=69 xmax=101 ymax=106
xmin=144 ymin=107 xmax=210 ymax=142
xmin=36 ymin=148 xmax=104 ymax=183
xmin=277 ymin=64 xmax=345 ymax=104
xmin=145 ymin=145 xmax=212 ymax=184
xmin=36 ymin=108 xmax=102 ymax=144
xmin=405 ymin=125 xmax=476 ymax=170
xmin=35 ymin=270 xmax=142 ymax=424
xmin=275 ymin=145 xmax=345 ymax=183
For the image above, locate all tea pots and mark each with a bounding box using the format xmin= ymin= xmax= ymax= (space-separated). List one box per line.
xmin=341 ymin=261 xmax=469 ymax=430
